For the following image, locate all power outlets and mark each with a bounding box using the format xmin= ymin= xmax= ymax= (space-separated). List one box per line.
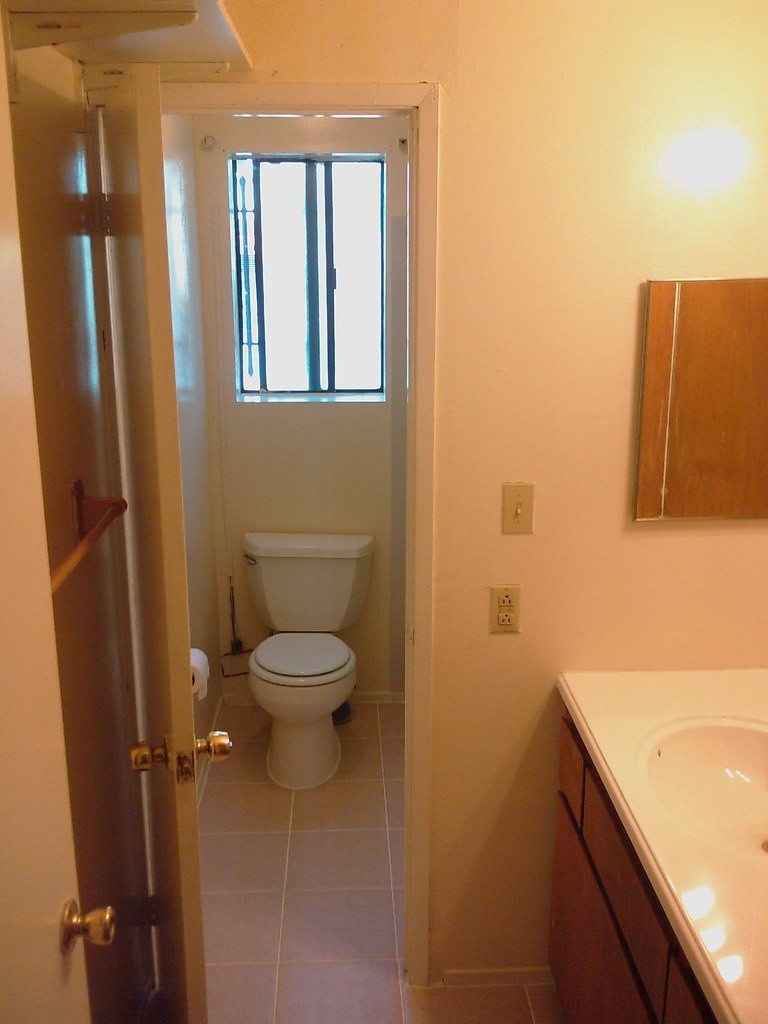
xmin=488 ymin=584 xmax=522 ymax=634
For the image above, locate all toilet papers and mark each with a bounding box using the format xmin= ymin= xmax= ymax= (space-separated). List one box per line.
xmin=190 ymin=647 xmax=211 ymax=702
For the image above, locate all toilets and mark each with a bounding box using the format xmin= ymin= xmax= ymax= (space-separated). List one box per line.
xmin=240 ymin=528 xmax=376 ymax=792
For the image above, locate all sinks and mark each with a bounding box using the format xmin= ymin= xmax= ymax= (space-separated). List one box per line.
xmin=635 ymin=711 xmax=768 ymax=865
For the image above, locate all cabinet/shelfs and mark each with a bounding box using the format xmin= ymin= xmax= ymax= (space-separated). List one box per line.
xmin=547 ymin=715 xmax=714 ymax=1024
